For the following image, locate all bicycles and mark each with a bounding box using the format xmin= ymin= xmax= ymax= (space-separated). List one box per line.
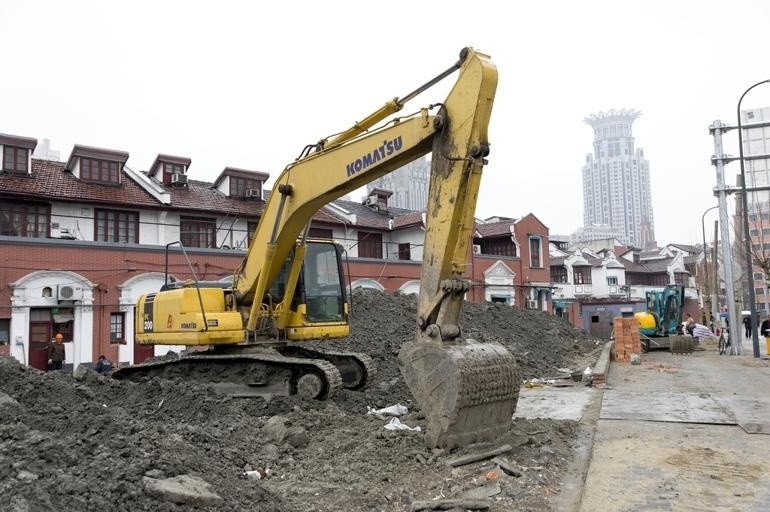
xmin=718 ymin=327 xmax=732 ymax=355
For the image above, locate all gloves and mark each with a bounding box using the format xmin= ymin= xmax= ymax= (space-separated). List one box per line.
xmin=48 ymin=359 xmax=52 ymax=364
xmin=62 ymin=360 xmax=66 ymax=364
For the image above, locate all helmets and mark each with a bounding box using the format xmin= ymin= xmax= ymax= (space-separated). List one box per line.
xmin=56 ymin=334 xmax=63 ymax=338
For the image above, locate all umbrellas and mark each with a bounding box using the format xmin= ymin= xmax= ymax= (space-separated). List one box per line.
xmin=720 ymin=312 xmax=728 ymax=317
xmin=741 ymin=311 xmax=751 ymax=315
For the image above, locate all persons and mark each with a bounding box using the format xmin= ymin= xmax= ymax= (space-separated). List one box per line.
xmin=721 ymin=305 xmax=727 ymax=311
xmin=685 ymin=313 xmax=696 ymax=336
xmin=744 ymin=315 xmax=751 ymax=339
xmin=761 ymin=314 xmax=770 ymax=355
xmin=702 ymin=312 xmax=706 ymax=325
xmin=725 ymin=317 xmax=728 ymax=327
xmin=96 ymin=355 xmax=112 ymax=373
xmin=709 ymin=312 xmax=715 ymax=333
xmin=47 ymin=333 xmax=65 ymax=370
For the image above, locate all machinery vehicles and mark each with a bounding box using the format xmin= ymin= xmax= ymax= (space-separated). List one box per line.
xmin=110 ymin=44 xmax=522 ymax=453
xmin=632 ymin=283 xmax=700 ymax=355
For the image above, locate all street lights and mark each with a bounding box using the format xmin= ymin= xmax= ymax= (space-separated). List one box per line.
xmin=701 ymin=204 xmax=719 ymax=284
xmin=738 ymin=79 xmax=770 ymax=358
